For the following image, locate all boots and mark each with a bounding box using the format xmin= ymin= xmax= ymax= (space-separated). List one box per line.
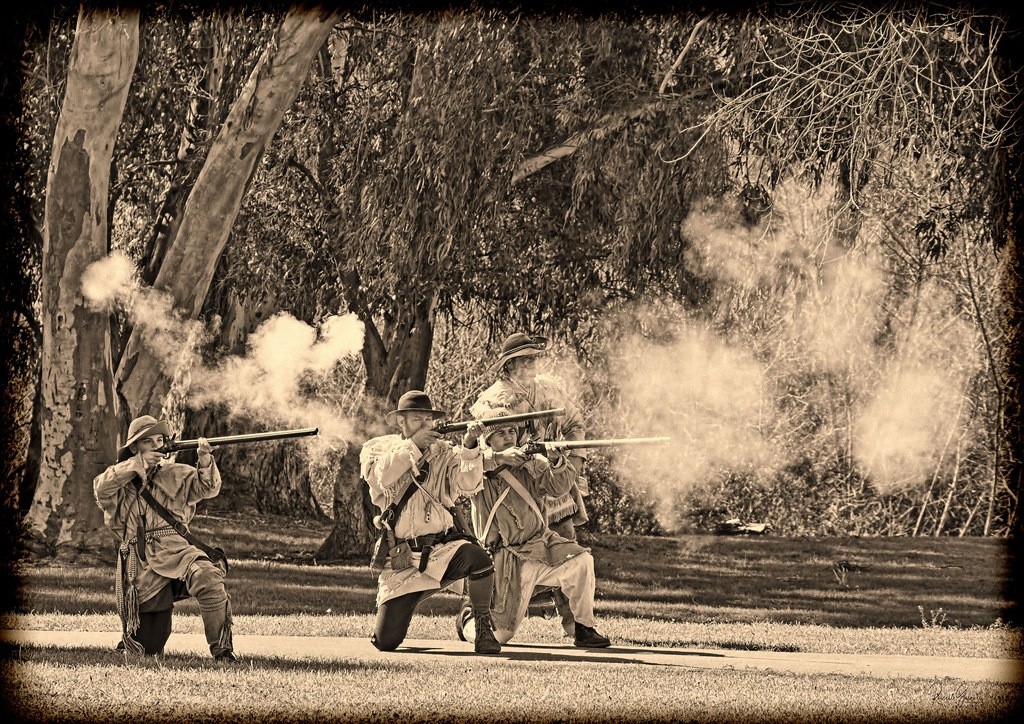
xmin=473 ymin=610 xmax=501 ymax=654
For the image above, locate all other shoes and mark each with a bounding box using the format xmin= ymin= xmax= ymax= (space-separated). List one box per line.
xmin=215 ymin=650 xmax=247 ymax=672
xmin=574 ymin=622 xmax=611 ymax=648
xmin=455 ymin=601 xmax=474 ymax=641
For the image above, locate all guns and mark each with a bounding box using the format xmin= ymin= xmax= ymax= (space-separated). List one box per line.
xmin=430 ymin=407 xmax=566 ymax=440
xmin=156 ymin=427 xmax=320 ymax=460
xmin=485 ymin=437 xmax=671 ymax=478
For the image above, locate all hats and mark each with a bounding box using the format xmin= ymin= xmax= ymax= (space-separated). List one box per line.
xmin=482 ymin=407 xmax=519 ymax=446
xmin=490 ymin=333 xmax=552 ymax=372
xmin=116 ymin=415 xmax=170 ymax=463
xmin=384 ymin=391 xmax=447 ymax=429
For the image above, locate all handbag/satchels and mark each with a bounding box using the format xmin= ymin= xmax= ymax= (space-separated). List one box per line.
xmin=207 ymin=548 xmax=228 ymax=576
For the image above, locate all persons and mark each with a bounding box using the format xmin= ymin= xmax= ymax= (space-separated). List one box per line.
xmin=91 ymin=417 xmax=236 ymax=664
xmin=358 ymin=389 xmax=503 ymax=656
xmin=469 ymin=331 xmax=588 ymax=639
xmin=456 ymin=408 xmax=612 ymax=650
